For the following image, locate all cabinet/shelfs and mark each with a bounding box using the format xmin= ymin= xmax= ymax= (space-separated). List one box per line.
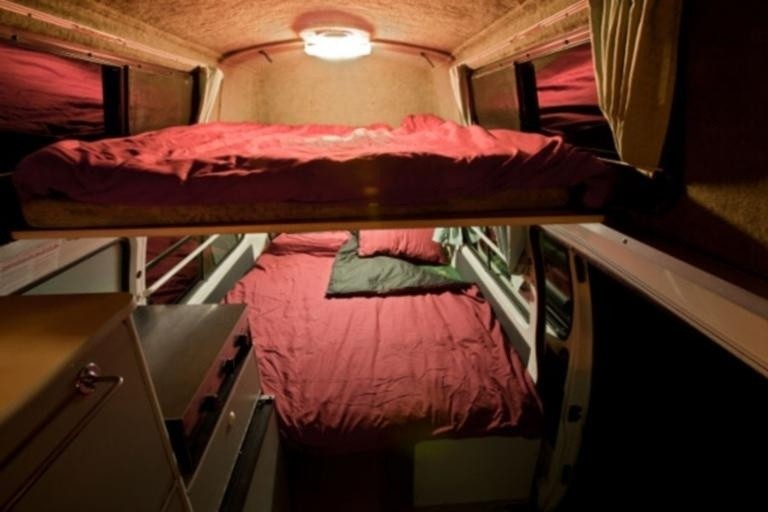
xmin=0 ymin=292 xmax=286 ymax=512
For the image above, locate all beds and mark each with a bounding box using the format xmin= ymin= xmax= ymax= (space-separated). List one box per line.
xmin=13 ymin=122 xmax=614 ymax=227
xmin=145 ymin=226 xmax=571 ymax=453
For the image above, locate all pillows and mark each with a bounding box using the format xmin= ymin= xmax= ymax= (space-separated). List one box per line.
xmin=271 ymin=230 xmax=353 ymax=256
xmin=358 ymin=228 xmax=447 ymax=266
